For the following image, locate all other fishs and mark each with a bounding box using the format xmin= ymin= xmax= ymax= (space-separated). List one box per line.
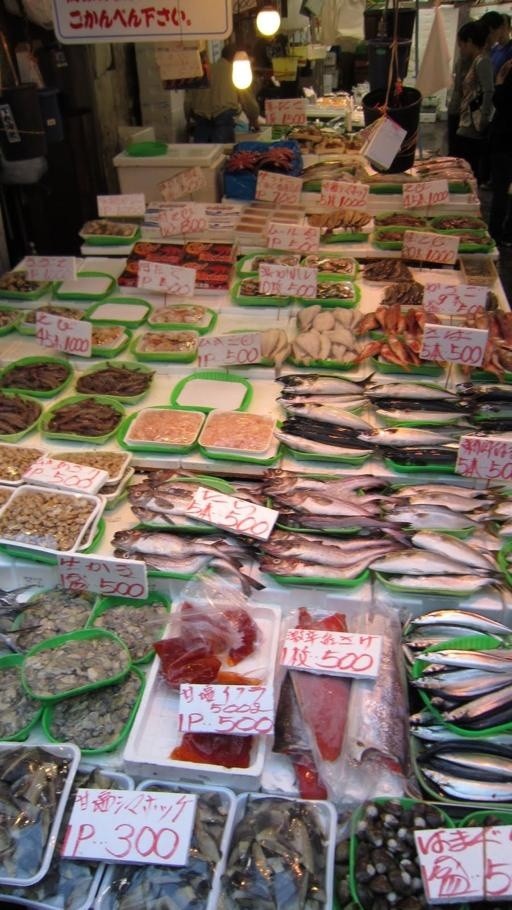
xmin=261 ymin=303 xmax=512 ymax=465
xmin=0 ymin=745 xmax=327 ymax=910
xmin=109 ymin=466 xmax=511 ymax=601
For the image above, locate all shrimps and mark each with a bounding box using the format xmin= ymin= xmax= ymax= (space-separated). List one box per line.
xmin=0 ymin=359 xmax=158 ymax=439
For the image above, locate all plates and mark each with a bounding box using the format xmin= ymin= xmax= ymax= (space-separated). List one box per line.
xmin=126 ymin=141 xmax=167 ymax=157
xmin=1 ymin=200 xmax=512 ymax=909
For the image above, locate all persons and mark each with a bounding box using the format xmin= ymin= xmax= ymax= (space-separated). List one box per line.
xmin=184 ymin=44 xmax=260 ymax=144
xmin=447 ymin=11 xmax=512 ymax=247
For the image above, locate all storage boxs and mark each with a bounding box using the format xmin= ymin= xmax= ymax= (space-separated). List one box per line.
xmin=114 ymin=142 xmax=225 ymax=203
xmin=0 ymin=84 xmax=48 ymax=161
xmin=363 ymin=9 xmax=418 ymax=82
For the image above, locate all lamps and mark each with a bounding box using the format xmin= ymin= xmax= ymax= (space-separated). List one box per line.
xmin=256 ymin=1 xmax=282 ymax=36
xmin=231 ymin=11 xmax=254 ymax=90
